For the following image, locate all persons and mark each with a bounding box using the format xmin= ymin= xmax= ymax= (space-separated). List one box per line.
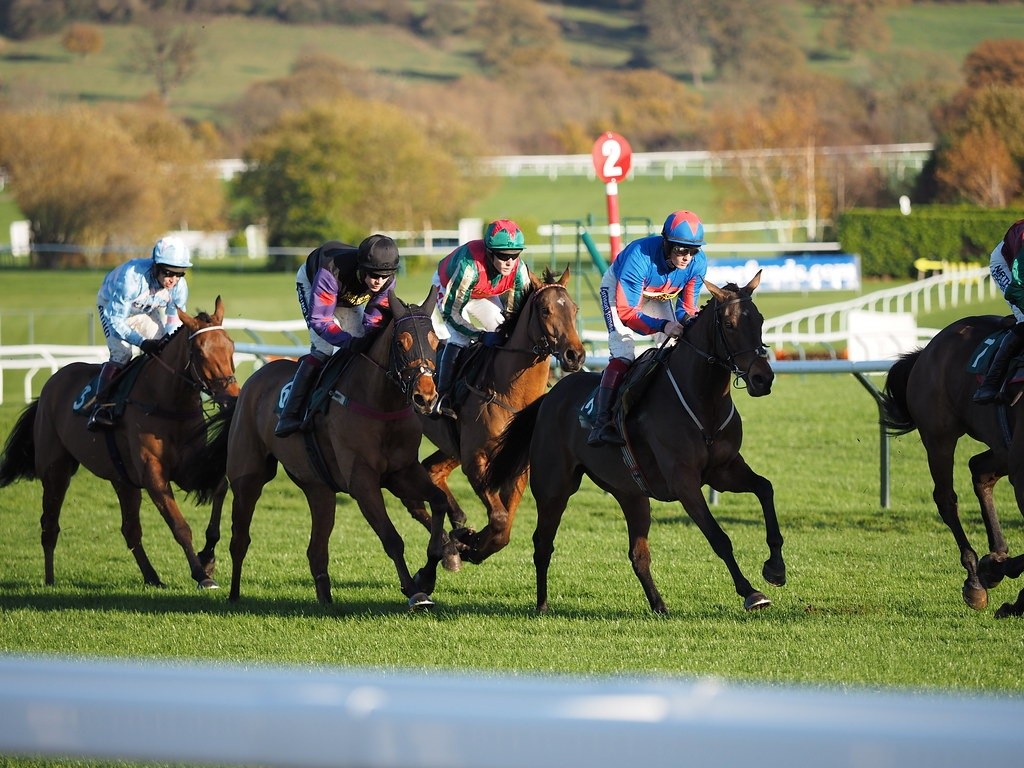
xmin=275 ymin=234 xmax=401 ymax=438
xmin=587 ymin=210 xmax=707 ymax=448
xmin=86 ymin=237 xmax=193 ymax=432
xmin=429 ymin=220 xmax=530 ymax=421
xmin=974 ymin=219 xmax=1024 ymax=405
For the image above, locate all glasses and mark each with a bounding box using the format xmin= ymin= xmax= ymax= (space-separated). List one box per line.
xmin=488 ymin=249 xmax=520 ymax=261
xmin=360 ymin=266 xmax=392 ymax=279
xmin=157 ymin=266 xmax=185 ymax=278
xmin=668 ymin=242 xmax=699 ymax=256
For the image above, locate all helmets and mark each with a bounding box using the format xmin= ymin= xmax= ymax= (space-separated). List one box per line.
xmin=358 ymin=235 xmax=399 ymax=275
xmin=153 ymin=236 xmax=193 ymax=268
xmin=661 ymin=211 xmax=706 ymax=245
xmin=485 ymin=220 xmax=526 ymax=249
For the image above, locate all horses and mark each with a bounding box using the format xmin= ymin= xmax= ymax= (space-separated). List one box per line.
xmin=0 ymin=261 xmax=786 ymax=615
xmin=877 ymin=315 xmax=1024 ymax=620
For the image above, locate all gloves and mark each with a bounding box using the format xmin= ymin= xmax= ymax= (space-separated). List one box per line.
xmin=349 ymin=337 xmax=363 ymax=352
xmin=478 ymin=330 xmax=506 ymax=349
xmin=140 ymin=339 xmax=160 ymax=354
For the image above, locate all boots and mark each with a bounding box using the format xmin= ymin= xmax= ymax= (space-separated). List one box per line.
xmin=429 ymin=342 xmax=467 ymax=421
xmin=972 ymin=330 xmax=1023 ymax=402
xmin=274 ymin=355 xmax=325 ymax=438
xmin=586 ymin=358 xmax=627 ymax=446
xmin=87 ymin=362 xmax=121 ymax=431
